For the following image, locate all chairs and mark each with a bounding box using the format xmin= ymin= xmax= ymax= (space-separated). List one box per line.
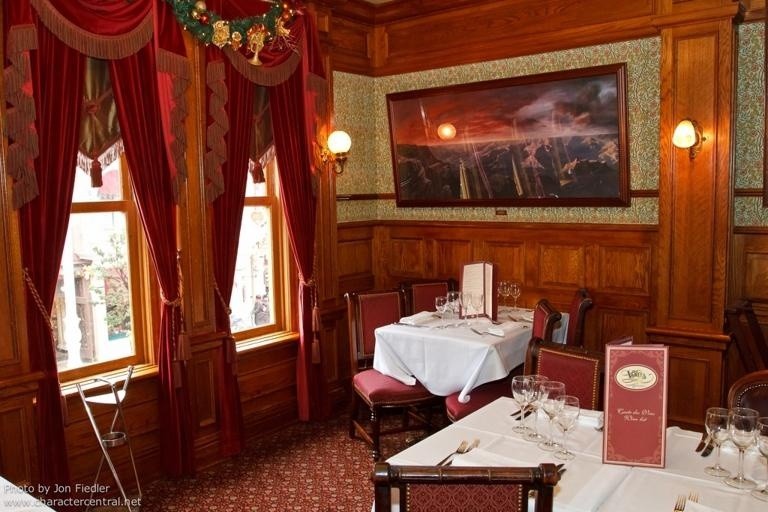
xmin=727 ymin=372 xmax=768 ymax=441
xmin=372 ymin=460 xmax=557 ymax=512
xmin=523 ymin=335 xmax=604 ymax=413
xmin=445 ymin=297 xmax=562 ymax=425
xmin=565 ymin=287 xmax=595 ymax=346
xmin=405 ymin=278 xmax=455 ymax=319
xmin=343 ymin=288 xmax=437 ymax=460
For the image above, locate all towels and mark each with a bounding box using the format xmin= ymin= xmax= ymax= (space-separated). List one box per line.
xmin=399 ymin=311 xmax=432 ymax=326
xmin=452 ymin=446 xmax=538 ymax=466
xmin=488 ymin=321 xmax=524 ymax=337
xmin=716 ymin=429 xmax=768 ymax=455
xmin=538 ymin=399 xmax=604 ymax=429
xmin=684 ymin=500 xmax=717 ymax=512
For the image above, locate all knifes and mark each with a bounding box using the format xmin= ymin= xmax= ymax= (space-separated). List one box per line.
xmin=509 ymin=410 xmax=520 ymax=417
xmin=701 ymin=438 xmax=714 ymax=457
xmin=515 ymin=407 xmax=535 ymax=422
xmin=694 ymin=429 xmax=709 ymax=453
xmin=394 ymin=322 xmax=428 ymax=328
xmin=483 ymin=332 xmax=505 ymax=339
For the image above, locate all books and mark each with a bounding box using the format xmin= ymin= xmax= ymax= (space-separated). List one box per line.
xmin=604 ymin=337 xmax=667 ymax=468
xmin=458 ymin=260 xmax=496 ymax=321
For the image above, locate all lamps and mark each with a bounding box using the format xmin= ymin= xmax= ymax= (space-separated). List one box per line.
xmin=672 ymin=119 xmax=702 ymax=161
xmin=320 ymin=131 xmax=353 ymax=176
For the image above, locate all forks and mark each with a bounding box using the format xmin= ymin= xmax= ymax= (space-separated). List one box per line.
xmin=443 ymin=438 xmax=482 ymax=466
xmin=688 ymin=490 xmax=701 ymax=501
xmin=433 ymin=439 xmax=469 ymax=466
xmin=673 ymin=493 xmax=688 ymax=511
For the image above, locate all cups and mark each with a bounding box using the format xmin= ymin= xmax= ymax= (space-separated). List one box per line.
xmin=458 ymin=290 xmax=472 ymax=329
xmin=523 ymin=374 xmax=550 ymax=443
xmin=703 ymin=406 xmax=734 ymax=478
xmin=508 ymin=282 xmax=521 ymax=313
xmin=446 ymin=292 xmax=460 ymax=330
xmin=553 ymin=394 xmax=581 ymax=463
xmin=536 ymin=381 xmax=566 ymax=452
xmin=750 ymin=417 xmax=768 ymax=503
xmin=723 ymin=407 xmax=762 ymax=492
xmin=435 ymin=296 xmax=448 ymax=329
xmin=511 ymin=375 xmax=536 ymax=435
xmin=497 ymin=279 xmax=511 ymax=314
xmin=471 ymin=288 xmax=484 ymax=325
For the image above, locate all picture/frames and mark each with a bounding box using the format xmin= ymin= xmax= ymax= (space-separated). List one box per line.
xmin=386 ymin=60 xmax=632 ymax=207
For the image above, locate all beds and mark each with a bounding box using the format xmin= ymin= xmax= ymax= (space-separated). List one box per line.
xmin=370 ymin=395 xmax=767 ymax=512
